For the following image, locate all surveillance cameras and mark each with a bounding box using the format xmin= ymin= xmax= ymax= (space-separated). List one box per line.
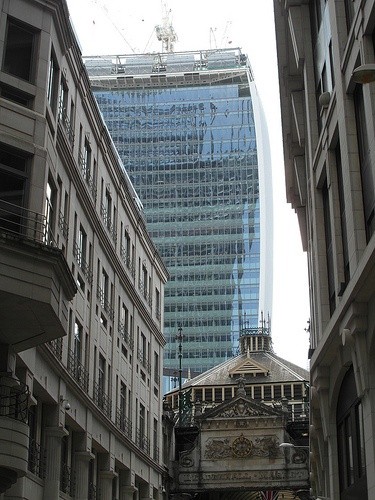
xmin=64 ymin=403 xmax=70 ymax=410
